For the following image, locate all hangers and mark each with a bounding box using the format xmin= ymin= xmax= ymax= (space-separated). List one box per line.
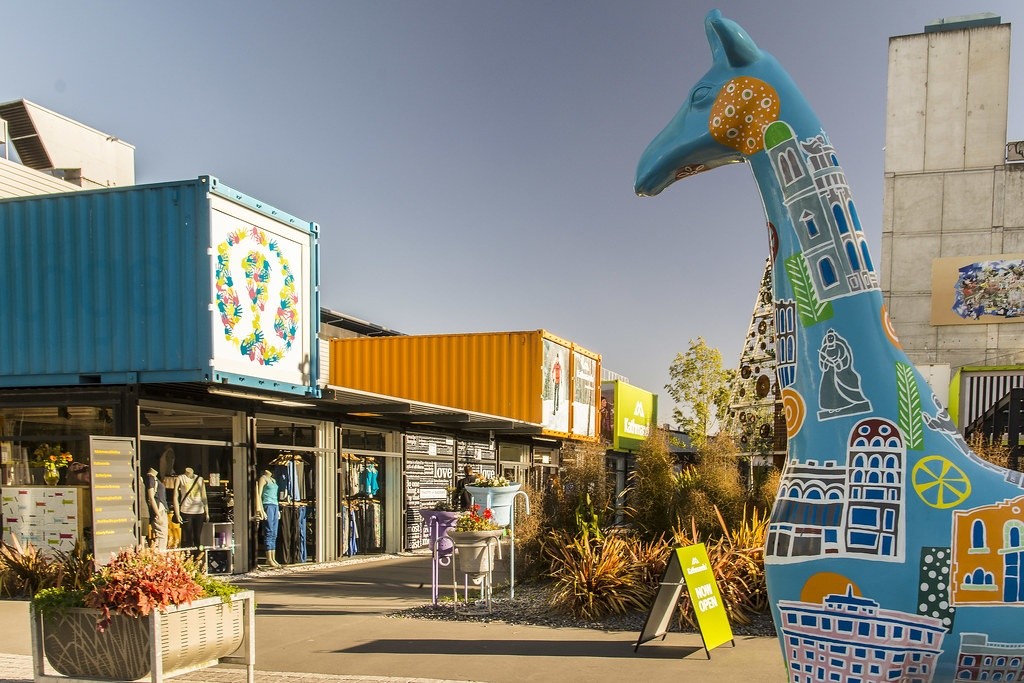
xmin=268 ymin=450 xmax=310 ymax=467
xmin=342 ymin=453 xmax=380 ymax=511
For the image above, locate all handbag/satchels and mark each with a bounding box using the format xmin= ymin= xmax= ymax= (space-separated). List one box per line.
xmin=171 ymin=510 xmax=182 ymax=524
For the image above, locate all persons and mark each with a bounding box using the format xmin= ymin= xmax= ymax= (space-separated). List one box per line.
xmin=140 ymin=443 xmax=210 ymax=563
xmin=598 ymin=396 xmax=613 ymax=447
xmin=552 ymin=352 xmax=563 ymax=416
xmin=453 ymin=465 xmax=486 ymax=511
xmin=249 ymin=470 xmax=281 ymax=567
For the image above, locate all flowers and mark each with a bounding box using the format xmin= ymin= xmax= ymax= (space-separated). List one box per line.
xmin=474 ymin=474 xmax=510 ymax=488
xmin=32 ymin=443 xmax=73 ymax=470
xmin=454 ymin=504 xmax=502 ymax=532
xmin=29 ymin=541 xmax=258 ymax=634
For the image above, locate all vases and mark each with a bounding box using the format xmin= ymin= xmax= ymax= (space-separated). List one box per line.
xmin=43 ymin=468 xmax=60 ymax=486
xmin=464 ymin=481 xmax=522 ymax=527
xmin=446 ymin=530 xmax=503 ymax=577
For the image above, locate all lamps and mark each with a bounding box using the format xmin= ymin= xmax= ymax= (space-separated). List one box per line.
xmin=140 ymin=413 xmax=151 ymax=427
xmin=58 ymin=407 xmax=72 ymax=420
xmin=99 ymin=410 xmax=113 ymax=424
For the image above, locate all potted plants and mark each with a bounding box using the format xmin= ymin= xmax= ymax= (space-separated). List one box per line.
xmin=418 ymin=501 xmax=461 ymax=557
